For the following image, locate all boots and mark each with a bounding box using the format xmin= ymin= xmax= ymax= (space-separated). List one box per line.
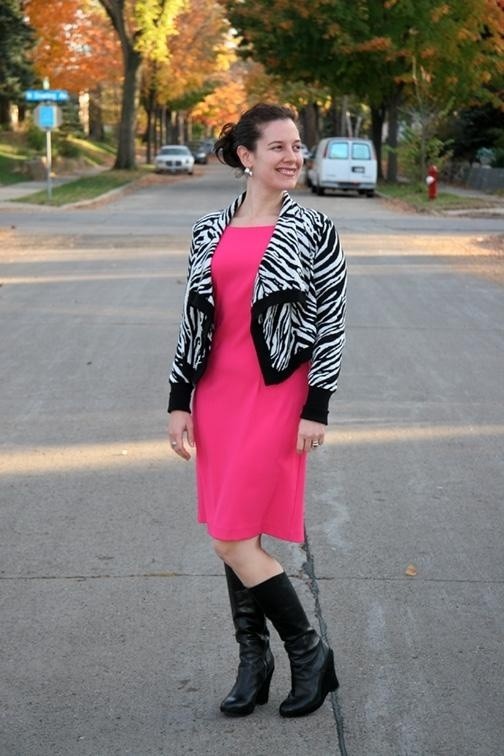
xmin=218 ymin=562 xmax=277 ymax=719
xmin=247 ymin=568 xmax=340 ymax=720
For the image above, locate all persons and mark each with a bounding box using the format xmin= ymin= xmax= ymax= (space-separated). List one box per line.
xmin=165 ymin=100 xmax=352 ymax=720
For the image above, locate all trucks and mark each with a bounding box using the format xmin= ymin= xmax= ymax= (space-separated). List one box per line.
xmin=313 ymin=136 xmax=383 ymax=197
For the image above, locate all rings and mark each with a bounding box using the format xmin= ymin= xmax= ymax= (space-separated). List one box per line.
xmin=170 ymin=441 xmax=177 ymax=448
xmin=311 ymin=442 xmax=320 ymax=448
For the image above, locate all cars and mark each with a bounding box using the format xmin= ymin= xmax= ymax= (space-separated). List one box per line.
xmin=301 ymin=144 xmax=317 ymax=186
xmin=152 ymin=135 xmax=219 ymax=175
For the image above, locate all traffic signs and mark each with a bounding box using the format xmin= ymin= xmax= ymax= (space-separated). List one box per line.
xmin=25 ymin=88 xmax=68 ymax=102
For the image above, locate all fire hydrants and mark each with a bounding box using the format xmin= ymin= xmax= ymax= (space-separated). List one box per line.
xmin=426 ymin=164 xmax=441 ymax=198
